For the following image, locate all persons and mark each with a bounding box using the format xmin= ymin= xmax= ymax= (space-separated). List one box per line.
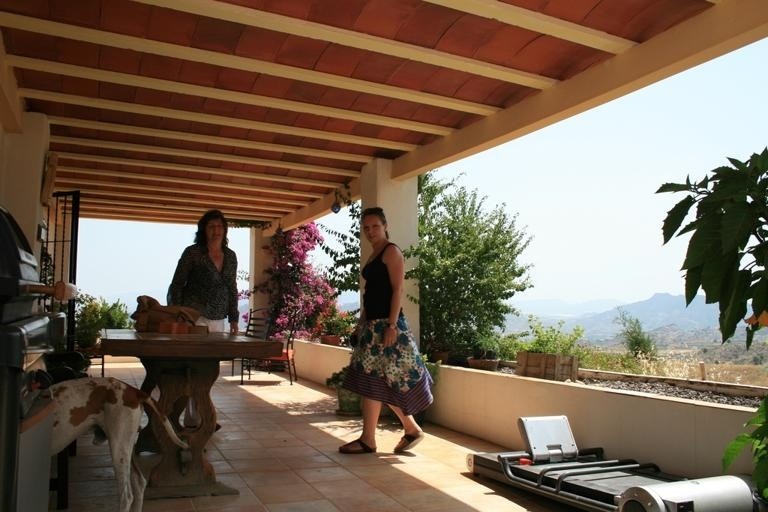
xmin=166 ymin=209 xmax=239 ymax=432
xmin=339 ymin=208 xmax=435 ymax=455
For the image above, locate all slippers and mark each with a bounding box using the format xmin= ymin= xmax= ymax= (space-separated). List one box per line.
xmin=393 ymin=430 xmax=426 ymax=453
xmin=338 ymin=437 xmax=377 ymax=454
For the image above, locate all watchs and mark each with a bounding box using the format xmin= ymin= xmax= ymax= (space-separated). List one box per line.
xmin=388 ymin=322 xmax=397 ymax=329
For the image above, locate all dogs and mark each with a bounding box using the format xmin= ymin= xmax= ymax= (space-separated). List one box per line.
xmin=52 ymin=377 xmax=193 ymax=512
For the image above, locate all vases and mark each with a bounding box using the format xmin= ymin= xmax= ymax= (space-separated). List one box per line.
xmin=318 ymin=332 xmax=342 ymax=346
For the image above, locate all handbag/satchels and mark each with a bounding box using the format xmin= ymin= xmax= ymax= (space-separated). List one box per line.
xmin=129 ymin=293 xmax=202 ymax=333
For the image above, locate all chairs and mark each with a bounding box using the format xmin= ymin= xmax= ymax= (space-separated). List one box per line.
xmin=237 ymin=306 xmax=303 ymax=387
xmin=230 ymin=305 xmax=274 ymax=378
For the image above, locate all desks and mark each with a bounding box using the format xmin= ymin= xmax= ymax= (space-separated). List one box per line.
xmin=71 ymin=341 xmax=106 ymax=380
xmin=97 ymin=327 xmax=284 ymax=501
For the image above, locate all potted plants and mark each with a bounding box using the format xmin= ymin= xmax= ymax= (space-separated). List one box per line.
xmin=516 ymin=312 xmax=588 ymax=383
xmin=466 ymin=332 xmax=502 ymax=372
xmin=428 ymin=335 xmax=451 ymax=364
xmin=411 ymin=351 xmax=444 ymax=429
xmin=324 ymin=360 xmax=361 ymax=416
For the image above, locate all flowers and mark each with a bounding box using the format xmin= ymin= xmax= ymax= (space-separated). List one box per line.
xmin=316 ymin=303 xmax=360 ymax=338
